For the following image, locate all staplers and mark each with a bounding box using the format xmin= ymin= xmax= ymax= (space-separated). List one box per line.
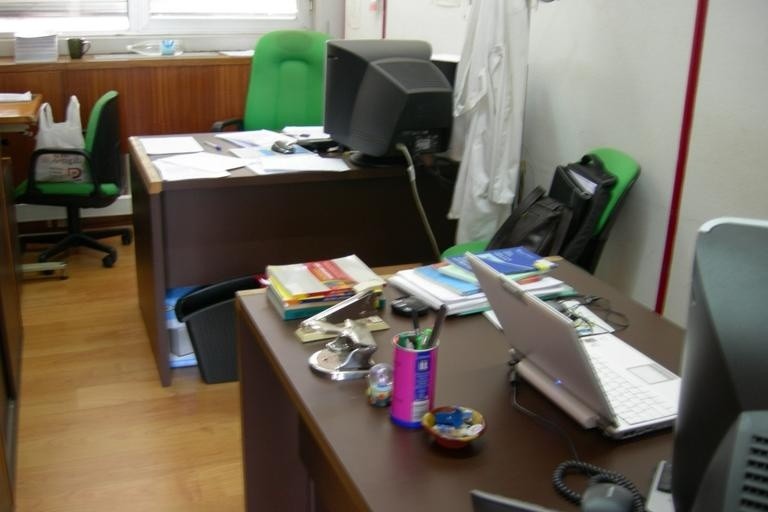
xmin=295 ymin=288 xmax=392 ymax=342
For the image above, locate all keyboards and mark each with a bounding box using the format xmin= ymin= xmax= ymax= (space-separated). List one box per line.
xmin=645 ymin=461 xmax=680 ymax=511
xmin=297 ymin=137 xmax=338 ymax=152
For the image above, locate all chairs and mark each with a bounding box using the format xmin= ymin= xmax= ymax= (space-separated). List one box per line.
xmin=208 ymin=28 xmax=341 ymax=135
xmin=437 ymin=147 xmax=643 ymax=281
xmin=9 ymin=86 xmax=138 ymax=279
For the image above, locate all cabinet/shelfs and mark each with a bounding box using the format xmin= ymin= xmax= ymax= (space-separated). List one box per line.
xmin=0 ymin=47 xmax=259 ymax=237
xmin=0 ymin=157 xmax=26 ymax=511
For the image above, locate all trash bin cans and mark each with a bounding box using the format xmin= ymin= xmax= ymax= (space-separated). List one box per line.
xmin=175 ymin=276 xmax=266 ymax=384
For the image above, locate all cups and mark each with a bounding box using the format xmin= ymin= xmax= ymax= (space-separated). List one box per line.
xmin=67 ymin=38 xmax=91 ymax=60
xmin=388 ymin=327 xmax=440 ymax=428
xmin=159 ymin=39 xmax=176 ymax=56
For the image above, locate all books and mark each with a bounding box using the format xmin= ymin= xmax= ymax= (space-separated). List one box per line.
xmin=13 ymin=33 xmax=59 ymax=64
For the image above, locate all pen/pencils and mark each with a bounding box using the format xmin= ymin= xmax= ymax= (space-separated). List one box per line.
xmin=400 ymin=310 xmax=432 ymax=349
xmin=204 ymin=139 xmax=221 ymax=151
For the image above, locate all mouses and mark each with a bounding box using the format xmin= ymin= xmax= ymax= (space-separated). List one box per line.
xmin=580 ymin=483 xmax=635 ymax=512
xmin=272 ymin=141 xmax=293 ymax=153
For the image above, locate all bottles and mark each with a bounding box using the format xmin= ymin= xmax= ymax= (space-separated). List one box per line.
xmin=127 ymin=40 xmax=184 ymax=57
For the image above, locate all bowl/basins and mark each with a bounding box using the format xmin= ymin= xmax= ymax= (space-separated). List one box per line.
xmin=416 ymin=402 xmax=488 ymax=448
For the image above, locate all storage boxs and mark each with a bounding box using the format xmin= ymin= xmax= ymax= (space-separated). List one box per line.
xmin=164 ymin=278 xmax=262 ymax=388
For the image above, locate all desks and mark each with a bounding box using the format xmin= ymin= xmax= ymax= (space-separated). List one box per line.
xmin=123 ymin=125 xmax=528 ymax=393
xmin=0 ymin=90 xmax=46 ymax=136
xmin=232 ymin=254 xmax=686 ymax=512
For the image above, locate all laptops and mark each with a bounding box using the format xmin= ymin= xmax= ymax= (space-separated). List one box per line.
xmin=466 ymin=249 xmax=682 ymax=441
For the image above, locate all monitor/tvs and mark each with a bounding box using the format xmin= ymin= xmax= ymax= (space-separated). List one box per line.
xmin=326 ymin=38 xmax=454 ymax=168
xmin=670 ymin=218 xmax=768 ymax=512
